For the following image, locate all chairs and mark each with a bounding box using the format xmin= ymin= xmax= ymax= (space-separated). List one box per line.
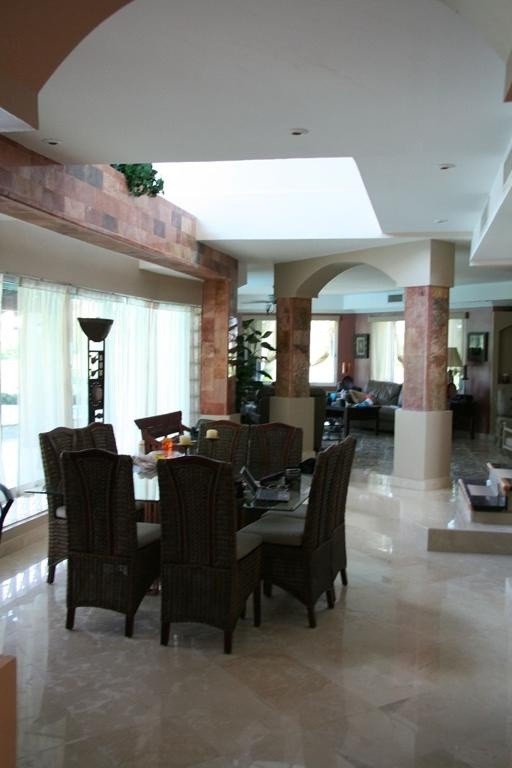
xmin=81 ymin=423 xmax=145 ymax=522
xmin=246 ymin=422 xmax=303 ymax=477
xmin=241 ymin=436 xmax=357 ymax=630
xmin=135 ymin=410 xmax=191 ymax=457
xmin=154 ymin=455 xmax=262 ymax=654
xmin=0 ymin=483 xmax=15 ymax=530
xmin=58 ymin=447 xmax=159 ymax=638
xmin=195 ymin=421 xmax=242 ymax=474
xmin=39 ymin=428 xmax=93 ymax=585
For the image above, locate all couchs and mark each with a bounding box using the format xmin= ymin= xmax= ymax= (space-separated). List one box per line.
xmin=351 ymin=379 xmax=474 ymax=438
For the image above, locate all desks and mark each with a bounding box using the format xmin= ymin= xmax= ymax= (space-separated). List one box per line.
xmin=24 ymin=462 xmax=314 ymax=512
xmin=0 ymin=654 xmax=16 ymax=768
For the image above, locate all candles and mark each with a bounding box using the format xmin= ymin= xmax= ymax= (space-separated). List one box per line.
xmin=178 ymin=435 xmax=191 ymax=444
xmin=205 ymin=429 xmax=218 ymax=438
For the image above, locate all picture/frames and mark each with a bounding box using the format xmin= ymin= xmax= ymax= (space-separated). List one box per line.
xmin=467 ymin=333 xmax=488 ymax=362
xmin=353 ymin=333 xmax=369 ymax=358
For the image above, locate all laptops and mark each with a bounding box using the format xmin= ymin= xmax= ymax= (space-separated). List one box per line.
xmin=239 ymin=464 xmax=290 ymax=502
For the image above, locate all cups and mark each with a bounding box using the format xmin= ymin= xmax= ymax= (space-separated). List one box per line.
xmin=286 ymin=467 xmax=302 ymax=492
xmin=162 ymin=438 xmax=174 ymax=458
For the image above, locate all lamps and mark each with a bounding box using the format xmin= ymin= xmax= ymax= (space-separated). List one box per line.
xmin=447 ymin=347 xmax=464 ymax=382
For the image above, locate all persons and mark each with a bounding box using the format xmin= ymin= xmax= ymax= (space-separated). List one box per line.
xmin=323 ymin=376 xmax=361 ymax=427
